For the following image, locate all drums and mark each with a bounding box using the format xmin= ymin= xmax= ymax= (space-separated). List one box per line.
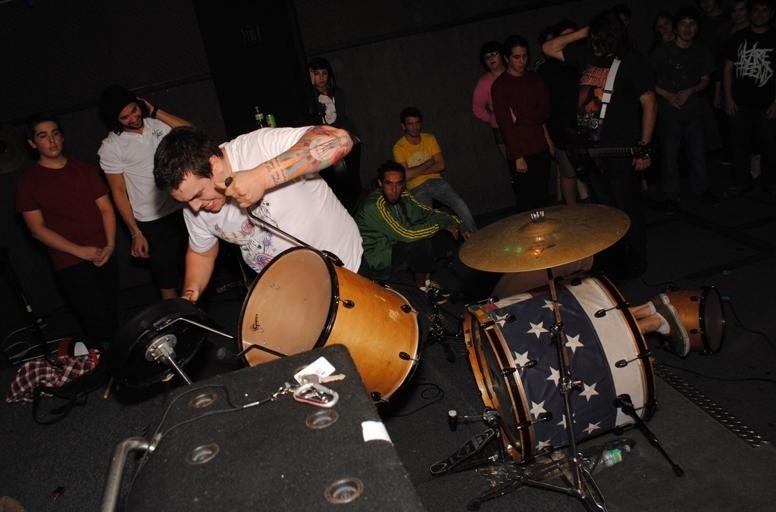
xmin=656 ymin=287 xmax=728 ymax=355
xmin=237 ymin=247 xmax=422 ymax=408
xmin=463 ymin=272 xmax=656 ymax=465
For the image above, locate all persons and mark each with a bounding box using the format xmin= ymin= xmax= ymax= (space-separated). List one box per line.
xmin=471 ymin=2 xmax=775 ymax=283
xmin=296 ymin=57 xmax=361 ymax=210
xmin=96 ymin=86 xmax=197 ymax=303
xmin=15 ymin=113 xmax=121 ymax=339
xmin=392 ymin=106 xmax=480 ymax=234
xmin=151 ymin=124 xmax=378 ymax=307
xmin=350 ymin=159 xmax=474 ymax=305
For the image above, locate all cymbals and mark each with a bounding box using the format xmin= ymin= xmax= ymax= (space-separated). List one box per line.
xmin=107 ymin=295 xmax=210 ymax=405
xmin=458 ymin=204 xmax=631 ymax=273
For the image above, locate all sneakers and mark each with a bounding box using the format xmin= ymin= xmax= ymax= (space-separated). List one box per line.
xmin=657 ymin=304 xmax=690 ymax=357
xmin=650 ymin=293 xmax=670 ymax=312
xmin=429 ymin=280 xmax=451 ymax=297
xmin=425 ymin=287 xmax=447 ymax=305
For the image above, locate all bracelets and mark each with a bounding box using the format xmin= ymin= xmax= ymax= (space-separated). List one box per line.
xmin=182 ymin=288 xmax=194 ymax=297
xmin=151 ymin=106 xmax=160 ymax=120
xmin=132 ymin=230 xmax=142 ymax=241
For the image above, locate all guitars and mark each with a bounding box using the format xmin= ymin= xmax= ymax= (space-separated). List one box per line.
xmin=563 ymin=134 xmax=659 ymax=171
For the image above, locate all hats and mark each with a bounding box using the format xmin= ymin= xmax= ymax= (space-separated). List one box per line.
xmin=99 ymin=84 xmax=136 ymax=120
